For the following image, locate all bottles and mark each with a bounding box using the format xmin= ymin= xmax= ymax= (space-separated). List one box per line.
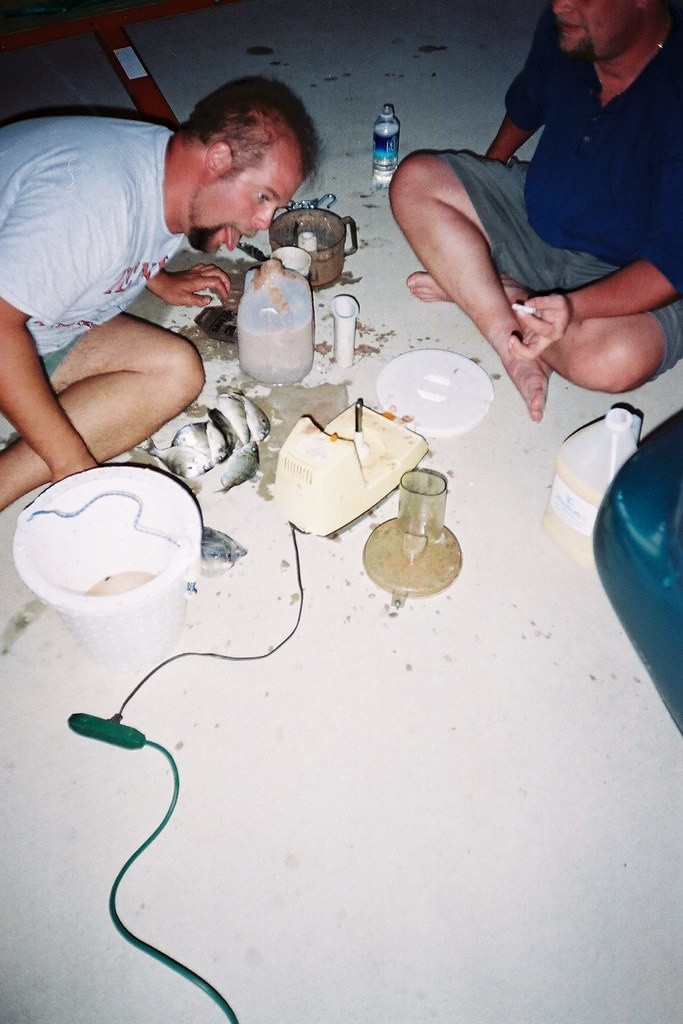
xmin=542 ymin=409 xmax=642 ymax=568
xmin=372 ymin=105 xmax=399 ymax=188
xmin=237 ymin=259 xmax=314 ymax=384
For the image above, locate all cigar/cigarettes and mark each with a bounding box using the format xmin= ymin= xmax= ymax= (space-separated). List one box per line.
xmin=511 ymin=303 xmax=536 ymax=314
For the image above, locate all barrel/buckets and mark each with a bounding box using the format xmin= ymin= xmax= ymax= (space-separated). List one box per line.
xmin=12 ymin=466 xmax=203 ymax=665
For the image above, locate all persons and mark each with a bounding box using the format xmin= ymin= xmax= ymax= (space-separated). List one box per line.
xmin=387 ymin=0 xmax=683 ymax=422
xmin=1 ymin=75 xmax=322 ymax=507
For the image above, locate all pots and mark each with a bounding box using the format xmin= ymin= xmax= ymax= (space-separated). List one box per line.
xmin=269 ymin=208 xmax=359 ymax=288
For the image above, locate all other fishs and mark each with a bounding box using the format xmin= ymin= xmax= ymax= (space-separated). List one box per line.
xmin=144 ymin=389 xmax=271 ymax=561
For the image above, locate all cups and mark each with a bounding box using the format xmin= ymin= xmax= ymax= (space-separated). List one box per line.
xmin=271 ymin=247 xmax=311 ymax=275
